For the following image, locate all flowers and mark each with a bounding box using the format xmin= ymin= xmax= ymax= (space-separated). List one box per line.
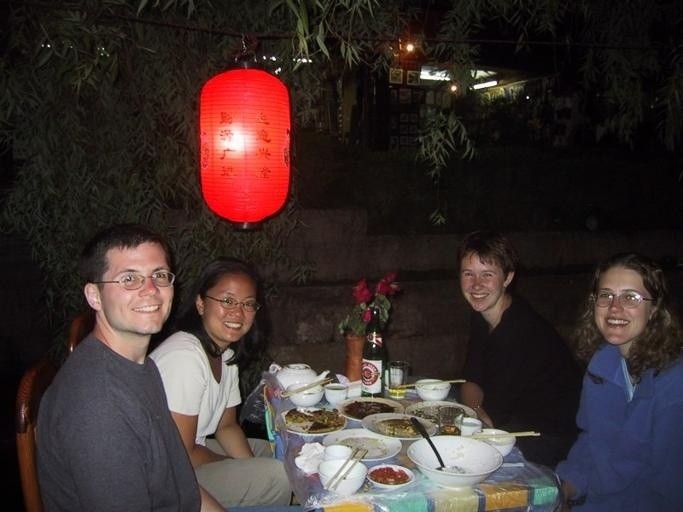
xmin=339 ymin=271 xmax=399 ymax=336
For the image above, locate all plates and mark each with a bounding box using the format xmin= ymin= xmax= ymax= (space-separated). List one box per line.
xmin=280 ymin=395 xmax=480 ymax=488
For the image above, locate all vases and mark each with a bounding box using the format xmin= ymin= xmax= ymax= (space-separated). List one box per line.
xmin=345 ymin=329 xmax=367 ymax=378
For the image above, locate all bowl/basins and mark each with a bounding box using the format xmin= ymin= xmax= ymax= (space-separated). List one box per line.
xmin=415 ymin=379 xmax=451 ymax=402
xmin=407 ymin=435 xmax=504 ymax=487
xmin=318 ymin=461 xmax=367 ymax=495
xmin=286 ymin=383 xmax=324 ymax=407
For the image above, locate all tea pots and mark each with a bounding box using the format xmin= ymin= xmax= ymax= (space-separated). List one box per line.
xmin=269 ymin=364 xmax=330 ymax=392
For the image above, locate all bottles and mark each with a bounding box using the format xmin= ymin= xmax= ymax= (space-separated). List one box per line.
xmin=359 ymin=309 xmax=386 ymax=397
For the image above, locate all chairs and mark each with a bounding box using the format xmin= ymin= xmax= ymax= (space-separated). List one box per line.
xmin=11 ymin=310 xmax=97 ymax=509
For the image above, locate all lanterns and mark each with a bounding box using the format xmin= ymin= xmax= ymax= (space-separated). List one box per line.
xmin=197 ymin=66 xmax=293 ymax=226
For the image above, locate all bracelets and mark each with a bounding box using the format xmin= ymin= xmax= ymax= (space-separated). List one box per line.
xmin=468 ymin=404 xmax=483 ymax=410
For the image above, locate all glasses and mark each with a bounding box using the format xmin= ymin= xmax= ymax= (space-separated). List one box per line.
xmin=205 ymin=295 xmax=262 ymax=312
xmin=591 ymin=290 xmax=657 ymax=308
xmin=90 ymin=270 xmax=175 ymax=290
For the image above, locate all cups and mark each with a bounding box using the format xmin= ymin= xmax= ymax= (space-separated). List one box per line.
xmin=388 ymin=361 xmax=407 ymax=399
xmin=438 ymin=405 xmax=464 ymax=437
xmin=325 ymin=383 xmax=347 ymax=403
xmin=461 ymin=418 xmax=482 ymax=437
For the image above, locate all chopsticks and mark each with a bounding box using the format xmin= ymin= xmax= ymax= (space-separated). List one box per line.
xmin=322 ymin=448 xmax=368 ymax=491
xmin=281 ymin=377 xmax=335 ymax=398
xmin=464 ymin=430 xmax=541 ymax=440
xmin=400 ymin=379 xmax=468 ymax=388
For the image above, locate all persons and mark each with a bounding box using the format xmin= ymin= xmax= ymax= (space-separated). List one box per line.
xmin=452 ymin=218 xmax=591 ymax=467
xmin=34 ymin=221 xmax=204 ymax=511
xmin=549 ymin=251 xmax=682 ymax=511
xmin=146 ymin=254 xmax=301 ymax=510
